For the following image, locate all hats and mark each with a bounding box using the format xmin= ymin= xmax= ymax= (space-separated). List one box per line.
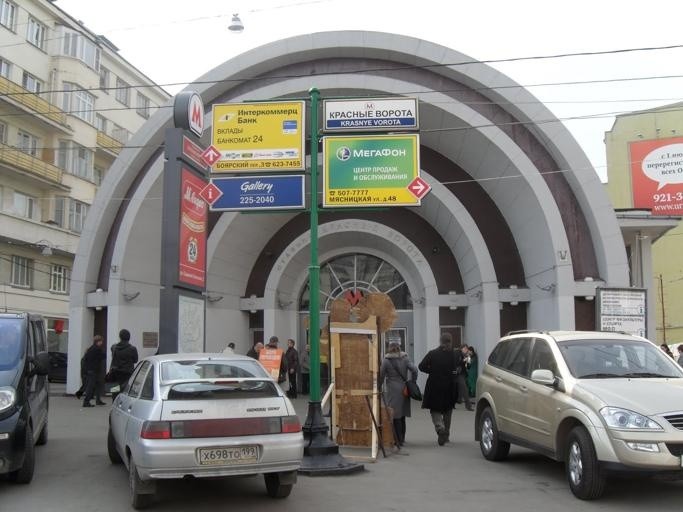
xmin=270 ymin=336 xmax=280 ymax=343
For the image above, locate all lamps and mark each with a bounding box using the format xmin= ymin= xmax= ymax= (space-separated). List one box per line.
xmin=35 ymin=239 xmax=53 ymax=256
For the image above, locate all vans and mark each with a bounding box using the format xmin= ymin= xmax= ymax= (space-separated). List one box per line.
xmin=0 ymin=312 xmax=52 ymax=485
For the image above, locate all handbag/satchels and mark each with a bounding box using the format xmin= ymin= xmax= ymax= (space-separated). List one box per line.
xmin=407 ymin=381 xmax=422 ymax=401
xmin=104 ymin=370 xmax=117 ymax=383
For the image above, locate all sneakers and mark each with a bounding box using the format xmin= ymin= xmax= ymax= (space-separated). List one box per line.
xmin=438 ymin=430 xmax=449 ymax=445
xmin=76 ymin=391 xmax=106 ymax=407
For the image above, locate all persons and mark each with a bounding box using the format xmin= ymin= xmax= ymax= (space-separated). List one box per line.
xmin=454 ymin=344 xmax=478 ymax=411
xmin=83 ymin=335 xmax=106 ymax=407
xmin=111 ymin=330 xmax=138 ymax=402
xmin=677 ymin=344 xmax=683 ymax=369
xmin=223 ymin=336 xmax=310 ymax=398
xmin=76 ymin=354 xmax=94 ymax=400
xmin=379 ymin=340 xmax=418 ymax=446
xmin=418 ymin=332 xmax=457 ymax=446
xmin=655 ymin=344 xmax=674 ymax=375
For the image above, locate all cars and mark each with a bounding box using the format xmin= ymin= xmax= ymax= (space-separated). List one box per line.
xmin=108 ymin=353 xmax=305 ymax=510
xmin=49 ymin=350 xmax=67 ymax=383
xmin=473 ymin=328 xmax=683 ymax=500
